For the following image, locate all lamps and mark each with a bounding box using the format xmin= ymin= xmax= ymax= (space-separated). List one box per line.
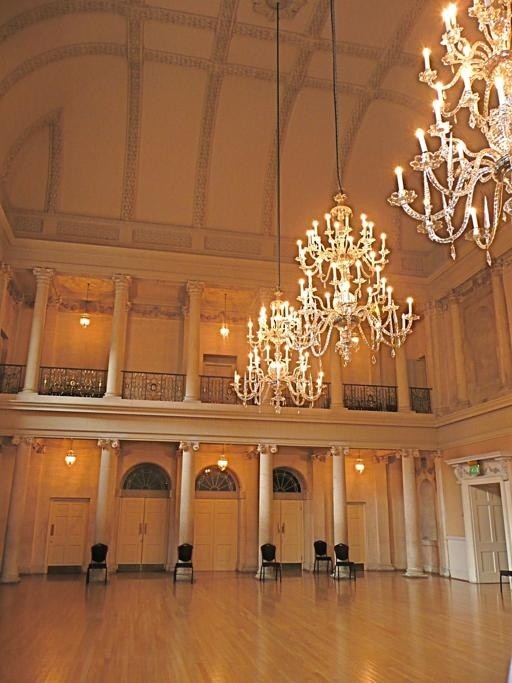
xmin=217 ymin=446 xmax=228 ymax=472
xmin=282 ymin=0 xmax=421 ymax=367
xmin=79 ymin=281 xmax=92 ymax=327
xmin=354 ymin=450 xmax=367 ymax=474
xmin=64 ymin=439 xmax=78 ymax=469
xmin=227 ymin=3 xmax=328 ymax=413
xmin=388 ymin=0 xmax=512 ymax=268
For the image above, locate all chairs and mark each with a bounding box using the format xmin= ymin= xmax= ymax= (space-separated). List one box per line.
xmin=258 ymin=543 xmax=287 ymax=582
xmin=83 ymin=540 xmax=113 ymax=587
xmin=172 ymin=541 xmax=198 ymax=584
xmin=312 ymin=540 xmax=335 ymax=578
xmin=333 ymin=542 xmax=357 ymax=580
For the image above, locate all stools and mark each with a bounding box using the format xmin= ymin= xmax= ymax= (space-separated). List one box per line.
xmin=499 ymin=568 xmax=511 ymax=592
